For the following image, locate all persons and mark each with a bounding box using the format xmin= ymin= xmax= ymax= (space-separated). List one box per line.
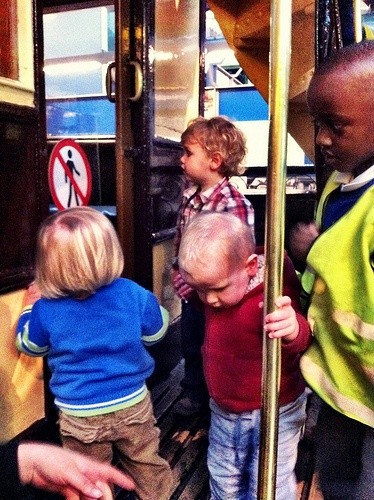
xmin=179 ymin=211 xmax=312 ymax=500
xmin=0 ymin=441 xmax=136 ymax=500
xmin=167 ymin=115 xmax=255 ymax=451
xmin=292 ymin=40 xmax=374 ymax=500
xmin=15 ymin=207 xmax=174 ymax=500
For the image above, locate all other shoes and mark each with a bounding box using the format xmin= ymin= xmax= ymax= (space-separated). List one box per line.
xmin=172 ymin=389 xmax=202 ymax=417
